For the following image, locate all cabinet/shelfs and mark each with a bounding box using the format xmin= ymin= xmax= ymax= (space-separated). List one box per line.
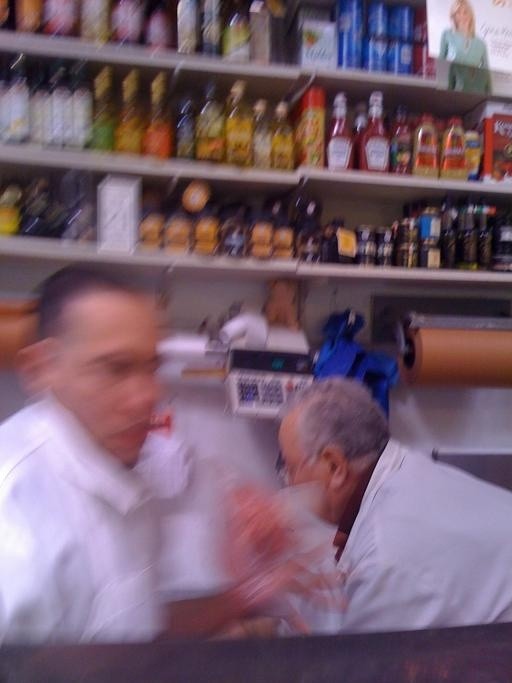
xmin=1 ymin=35 xmax=512 ymax=283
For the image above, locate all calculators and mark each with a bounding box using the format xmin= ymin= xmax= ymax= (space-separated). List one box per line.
xmin=224 ymin=350 xmax=315 ymax=419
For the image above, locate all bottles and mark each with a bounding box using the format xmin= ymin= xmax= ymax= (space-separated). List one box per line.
xmin=179 ymin=79 xmax=295 ymax=171
xmin=0 ymin=66 xmax=94 ymax=148
xmin=92 ymin=64 xmax=177 ymax=158
xmin=353 ymin=90 xmax=391 ymax=173
xmin=384 ymin=102 xmax=414 ymax=175
xmin=396 ymin=207 xmax=442 ymax=268
xmin=435 ymin=115 xmax=469 ymax=180
xmin=324 ymin=91 xmax=355 ymax=170
xmin=410 ymin=112 xmax=440 ymax=177
xmin=334 ymin=0 xmax=416 ymax=75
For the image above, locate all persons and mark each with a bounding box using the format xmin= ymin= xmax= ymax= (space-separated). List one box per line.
xmin=437 ymin=0 xmax=491 ymax=68
xmin=0 ymin=261 xmax=350 ymax=644
xmin=212 ymin=374 xmax=512 ymax=642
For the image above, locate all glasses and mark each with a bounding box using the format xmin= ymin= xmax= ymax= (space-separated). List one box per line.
xmin=276 ymin=450 xmax=323 ymax=485
